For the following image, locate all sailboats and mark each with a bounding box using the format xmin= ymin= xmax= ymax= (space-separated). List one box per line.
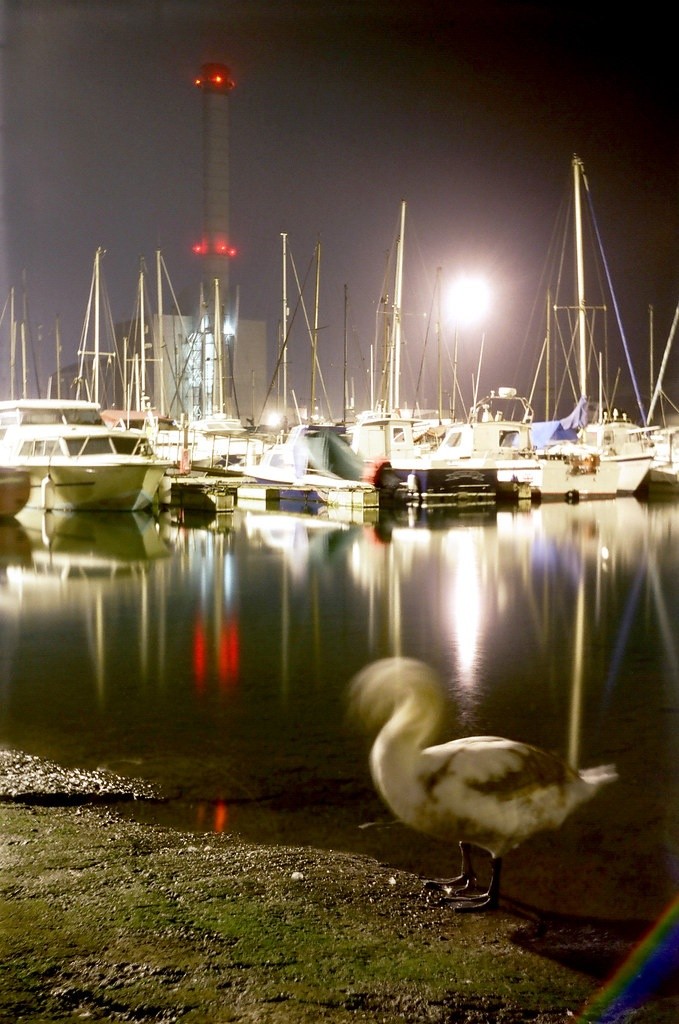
xmin=1 ymin=152 xmax=679 ymax=522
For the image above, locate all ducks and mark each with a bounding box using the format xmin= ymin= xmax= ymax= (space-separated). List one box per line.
xmin=353 ymin=658 xmax=626 ymax=913
